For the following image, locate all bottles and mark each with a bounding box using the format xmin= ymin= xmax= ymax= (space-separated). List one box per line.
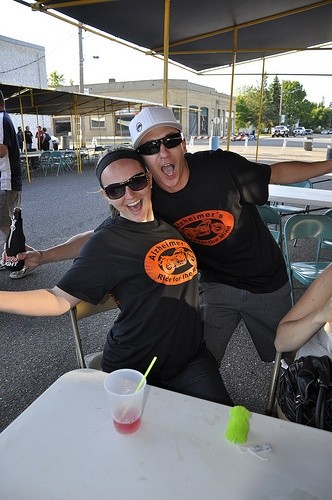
xmin=4 ymin=207 xmax=26 ymax=272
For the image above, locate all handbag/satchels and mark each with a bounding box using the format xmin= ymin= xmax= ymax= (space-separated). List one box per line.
xmin=277 ymin=355 xmax=331 ymax=431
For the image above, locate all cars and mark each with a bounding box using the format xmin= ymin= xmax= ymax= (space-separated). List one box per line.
xmin=305 ymin=129 xmax=313 ymax=135
xmin=320 ymin=129 xmax=332 ymax=134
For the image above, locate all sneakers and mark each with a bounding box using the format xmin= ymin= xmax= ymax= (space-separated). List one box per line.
xmin=9 ymin=265 xmax=31 ymax=279
xmin=0 ymin=260 xmax=6 ymax=271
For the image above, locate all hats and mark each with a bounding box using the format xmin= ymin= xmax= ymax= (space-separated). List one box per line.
xmin=128 ymin=105 xmax=182 ymax=149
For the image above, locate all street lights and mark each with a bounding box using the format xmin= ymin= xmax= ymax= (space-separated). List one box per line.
xmin=278 ymin=85 xmax=295 ymax=126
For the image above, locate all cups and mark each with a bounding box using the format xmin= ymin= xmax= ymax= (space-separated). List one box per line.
xmin=103 ymin=368 xmax=147 ymax=435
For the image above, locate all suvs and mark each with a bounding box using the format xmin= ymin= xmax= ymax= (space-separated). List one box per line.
xmin=292 ymin=127 xmax=306 ymax=137
xmin=271 ymin=125 xmax=290 ymax=138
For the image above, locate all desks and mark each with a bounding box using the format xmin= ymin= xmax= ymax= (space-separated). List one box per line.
xmin=20 ymin=154 xmax=41 ymax=180
xmin=266 ymin=184 xmax=332 ymax=207
xmin=0 ymin=368 xmax=332 ymax=500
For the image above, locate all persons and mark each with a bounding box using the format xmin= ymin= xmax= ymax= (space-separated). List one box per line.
xmin=2 ymin=102 xmax=332 ymax=374
xmin=0 ymin=148 xmax=231 ymax=408
xmin=272 ymin=263 xmax=332 ymax=426
xmin=15 ymin=124 xmax=54 ymax=154
xmin=0 ymin=91 xmax=41 ymax=280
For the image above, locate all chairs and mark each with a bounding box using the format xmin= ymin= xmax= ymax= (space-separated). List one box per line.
xmin=283 ymin=214 xmax=332 ymax=309
xmin=70 ymin=293 xmax=118 ymax=370
xmin=257 ymin=205 xmax=284 ymax=253
xmin=275 ymin=179 xmax=313 ymax=247
xmin=39 ymin=147 xmax=105 ymax=177
xmin=316 ymin=209 xmax=332 ymax=262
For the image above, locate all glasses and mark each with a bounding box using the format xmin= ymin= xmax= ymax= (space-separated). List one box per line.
xmin=137 ymin=133 xmax=183 ymax=155
xmin=104 ymin=171 xmax=149 ymax=199
